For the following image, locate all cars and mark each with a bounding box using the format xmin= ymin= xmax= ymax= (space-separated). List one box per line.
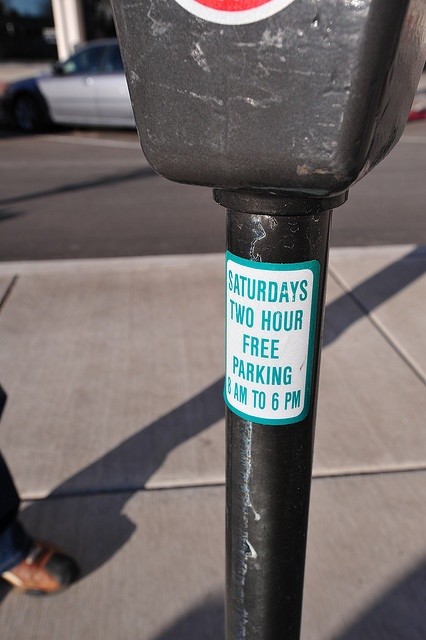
xmin=5 ymin=39 xmax=135 ymax=131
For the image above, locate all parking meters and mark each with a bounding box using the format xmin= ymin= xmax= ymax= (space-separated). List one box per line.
xmin=108 ymin=0 xmax=426 ymax=640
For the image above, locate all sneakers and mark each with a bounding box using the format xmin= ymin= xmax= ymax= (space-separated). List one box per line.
xmin=3 ymin=539 xmax=80 ymax=600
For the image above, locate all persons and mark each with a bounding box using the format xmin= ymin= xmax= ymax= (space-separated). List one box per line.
xmin=0 ymin=384 xmax=78 ymax=597
